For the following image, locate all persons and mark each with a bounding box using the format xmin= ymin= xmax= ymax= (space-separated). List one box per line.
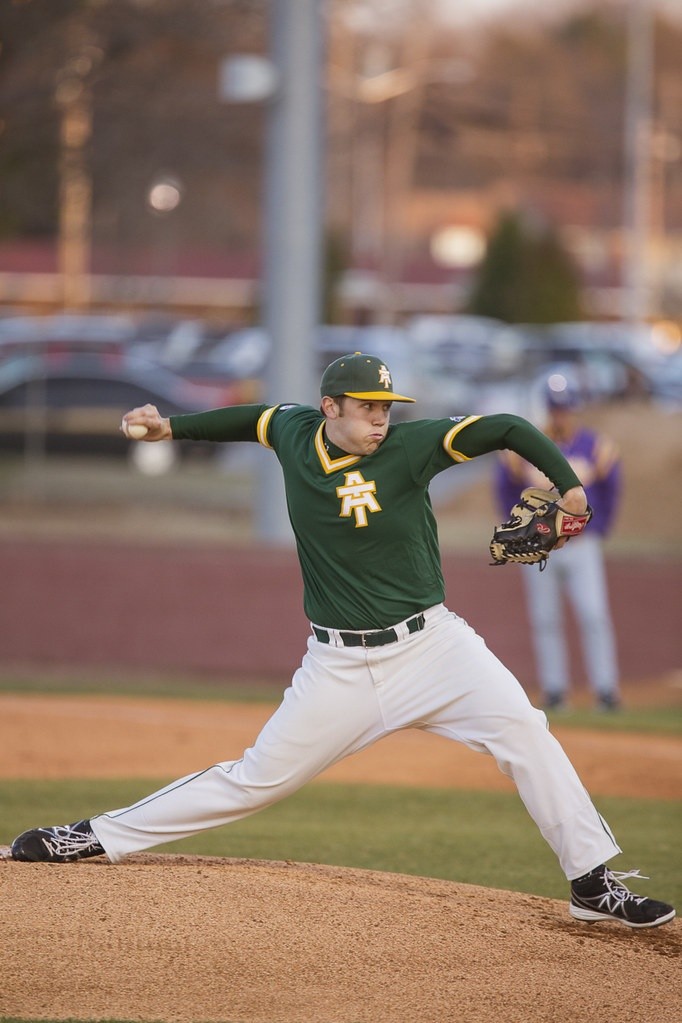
xmin=11 ymin=352 xmax=677 ymax=928
xmin=493 ymin=370 xmax=620 ymax=713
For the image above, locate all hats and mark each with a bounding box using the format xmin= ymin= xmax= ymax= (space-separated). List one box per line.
xmin=321 ymin=352 xmax=416 ymax=403
xmin=544 ymin=373 xmax=574 ymax=406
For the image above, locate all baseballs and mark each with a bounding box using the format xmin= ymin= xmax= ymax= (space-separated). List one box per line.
xmin=121 ymin=416 xmax=148 ymax=440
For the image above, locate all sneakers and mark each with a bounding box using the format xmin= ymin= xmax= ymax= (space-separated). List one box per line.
xmin=11 ymin=818 xmax=106 ymax=862
xmin=570 ymin=862 xmax=676 ymax=930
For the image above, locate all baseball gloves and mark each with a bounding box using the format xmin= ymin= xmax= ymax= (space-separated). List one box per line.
xmin=489 ymin=486 xmax=594 ymax=566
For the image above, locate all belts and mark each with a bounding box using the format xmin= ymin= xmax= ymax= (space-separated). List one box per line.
xmin=312 ymin=614 xmax=424 ymax=649
